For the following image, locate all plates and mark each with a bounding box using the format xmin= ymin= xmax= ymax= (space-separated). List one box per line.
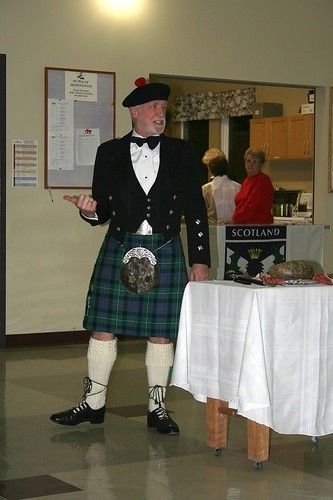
xmin=280 ymin=279 xmax=321 ymax=287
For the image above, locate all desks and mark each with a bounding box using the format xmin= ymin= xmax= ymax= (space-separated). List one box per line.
xmin=169 ymin=280 xmax=333 ymax=473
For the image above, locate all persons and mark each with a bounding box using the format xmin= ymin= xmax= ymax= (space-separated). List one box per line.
xmin=217 ymin=148 xmax=275 ymax=224
xmin=202 ymin=148 xmax=226 ymax=182
xmin=50 ymin=427 xmax=173 ymax=500
xmin=202 ymin=157 xmax=242 ymax=225
xmin=50 ymin=78 xmax=211 ymax=434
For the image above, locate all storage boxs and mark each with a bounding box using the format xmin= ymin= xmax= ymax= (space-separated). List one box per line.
xmin=251 ymin=102 xmax=283 ymax=118
xmin=297 ymin=103 xmax=315 ymax=115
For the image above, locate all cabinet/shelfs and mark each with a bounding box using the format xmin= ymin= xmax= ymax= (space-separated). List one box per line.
xmin=288 ymin=114 xmax=314 ymax=160
xmin=250 ymin=115 xmax=288 ymax=161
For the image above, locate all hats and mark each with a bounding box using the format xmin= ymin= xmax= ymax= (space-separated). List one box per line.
xmin=122 ymin=83 xmax=170 ymax=108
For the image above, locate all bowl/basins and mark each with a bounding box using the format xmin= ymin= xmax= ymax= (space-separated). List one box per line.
xmin=296 ymin=212 xmax=312 ymax=218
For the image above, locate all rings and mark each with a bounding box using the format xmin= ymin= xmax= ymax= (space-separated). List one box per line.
xmin=88 ymin=201 xmax=92 ymax=204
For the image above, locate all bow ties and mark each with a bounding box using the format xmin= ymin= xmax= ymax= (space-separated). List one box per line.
xmin=128 ymin=135 xmax=160 ymax=150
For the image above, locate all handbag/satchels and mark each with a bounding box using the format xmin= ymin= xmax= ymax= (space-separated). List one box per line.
xmin=120 ymin=245 xmax=160 ymax=295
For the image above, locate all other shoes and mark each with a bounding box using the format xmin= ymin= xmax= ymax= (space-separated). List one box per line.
xmin=49 ymin=398 xmax=106 ymax=426
xmin=146 ymin=407 xmax=180 ymax=435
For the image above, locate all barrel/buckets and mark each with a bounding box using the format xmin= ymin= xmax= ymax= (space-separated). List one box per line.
xmin=272 ymin=189 xmax=297 ymax=217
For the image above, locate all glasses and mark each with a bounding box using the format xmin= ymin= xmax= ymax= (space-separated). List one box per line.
xmin=245 ymin=159 xmax=257 ymax=164
